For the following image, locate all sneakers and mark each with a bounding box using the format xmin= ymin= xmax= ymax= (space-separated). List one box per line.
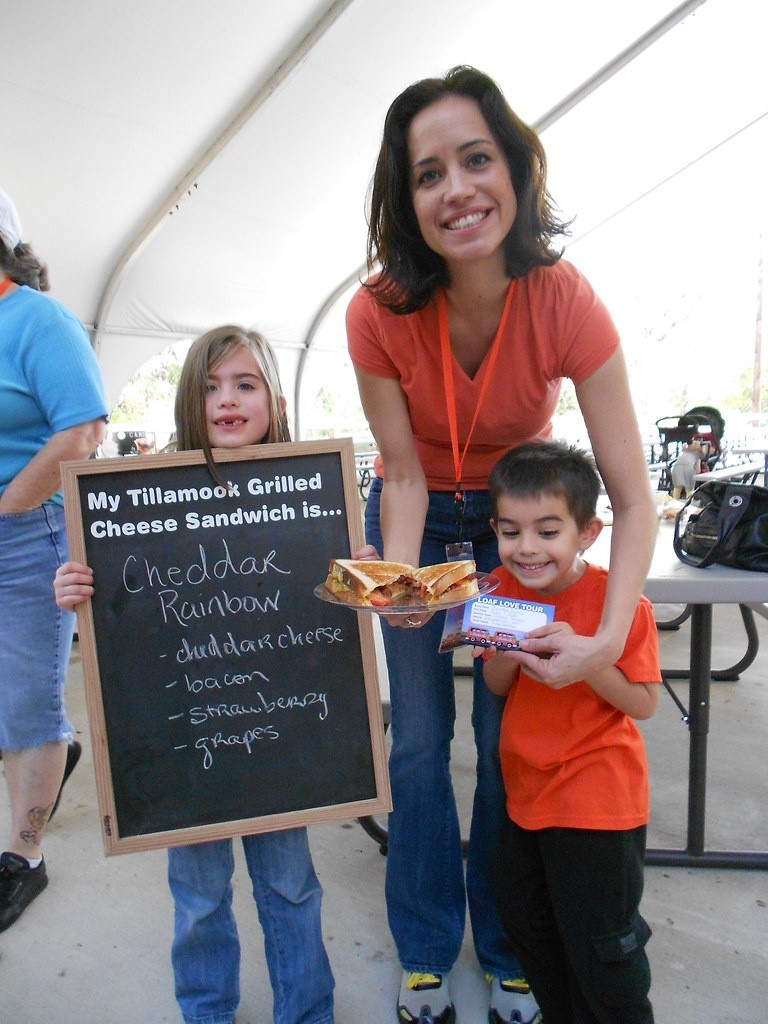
xmin=485 ymin=972 xmax=543 ymax=1024
xmin=396 ymin=969 xmax=456 ymax=1024
xmin=0 ymin=851 xmax=48 ymax=933
xmin=47 ymin=741 xmax=81 ymax=824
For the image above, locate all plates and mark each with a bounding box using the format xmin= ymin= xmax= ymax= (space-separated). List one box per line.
xmin=314 ymin=571 xmax=501 ymax=614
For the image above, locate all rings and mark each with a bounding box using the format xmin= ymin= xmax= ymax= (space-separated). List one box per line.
xmin=407 ymin=619 xmax=421 ymax=628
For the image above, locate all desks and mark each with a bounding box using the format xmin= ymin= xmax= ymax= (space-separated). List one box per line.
xmin=354 ymin=451 xmax=378 ymax=478
xmin=581 ymin=491 xmax=768 ymax=869
xmin=732 ymin=443 xmax=768 ymax=488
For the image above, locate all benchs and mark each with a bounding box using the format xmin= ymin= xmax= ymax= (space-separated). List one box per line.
xmin=356 ymin=465 xmax=374 ymax=496
xmin=692 ymin=461 xmax=765 ymax=482
xmin=731 ymin=603 xmax=768 ymax=674
xmin=356 ymin=456 xmax=378 ymax=486
xmin=358 ymin=612 xmax=392 ymax=832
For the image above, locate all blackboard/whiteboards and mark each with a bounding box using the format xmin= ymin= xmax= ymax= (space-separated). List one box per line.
xmin=58 ymin=436 xmax=394 ymax=858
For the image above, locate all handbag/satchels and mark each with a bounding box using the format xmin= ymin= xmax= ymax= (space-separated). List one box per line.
xmin=673 ymin=480 xmax=768 ymax=572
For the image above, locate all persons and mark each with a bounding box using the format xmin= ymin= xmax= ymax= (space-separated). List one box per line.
xmin=671 ymin=443 xmax=705 ymax=500
xmin=346 ymin=65 xmax=653 ymax=1024
xmin=0 ymin=189 xmax=110 ymax=932
xmin=53 ymin=327 xmax=384 ymax=1024
xmin=471 ymin=442 xmax=659 ymax=1024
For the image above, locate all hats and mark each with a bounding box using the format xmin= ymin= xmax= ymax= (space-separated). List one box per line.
xmin=0 ymin=188 xmax=24 ymax=250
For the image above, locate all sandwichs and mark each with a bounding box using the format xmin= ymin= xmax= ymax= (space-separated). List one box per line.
xmin=406 ymin=560 xmax=479 ymax=605
xmin=323 ymin=558 xmax=416 ymax=607
